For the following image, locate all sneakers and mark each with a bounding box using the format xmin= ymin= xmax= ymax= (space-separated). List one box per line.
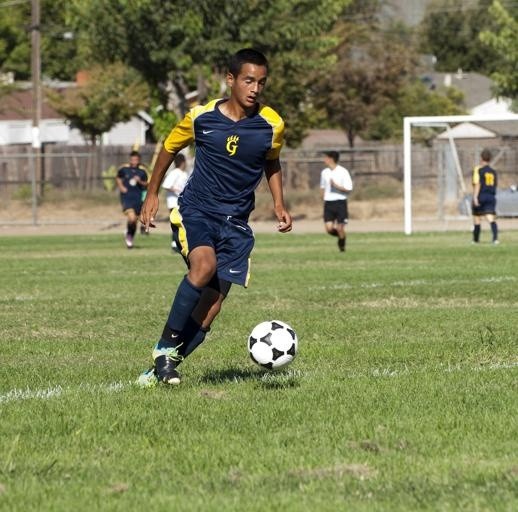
xmin=135 ymin=344 xmax=184 ymax=388
xmin=125 ymin=233 xmax=133 ymax=247
xmin=471 ymin=239 xmax=499 ymax=244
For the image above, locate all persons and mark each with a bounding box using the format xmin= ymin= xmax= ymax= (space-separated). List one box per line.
xmin=161 ymin=153 xmax=190 ymax=250
xmin=115 ymin=150 xmax=151 ymax=248
xmin=140 ymin=191 xmax=156 ymax=234
xmin=136 ymin=48 xmax=292 ymax=388
xmin=471 ymin=150 xmax=499 ymax=244
xmin=320 ymin=151 xmax=353 ymax=251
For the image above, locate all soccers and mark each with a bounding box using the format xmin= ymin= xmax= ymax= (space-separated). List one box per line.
xmin=248 ymin=320 xmax=298 ymax=369
